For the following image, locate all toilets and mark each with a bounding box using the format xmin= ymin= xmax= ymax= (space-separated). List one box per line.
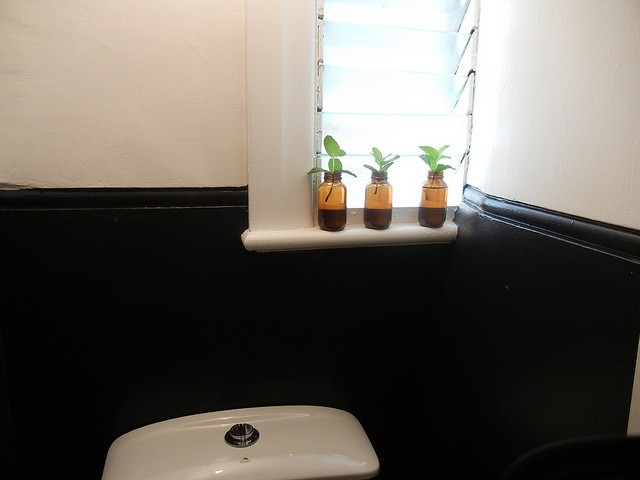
xmin=100 ymin=405 xmax=382 ymax=480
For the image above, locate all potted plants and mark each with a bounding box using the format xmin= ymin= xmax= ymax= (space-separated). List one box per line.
xmin=364 ymin=146 xmax=400 ymax=231
xmin=307 ymin=135 xmax=357 ymax=232
xmin=419 ymin=145 xmax=455 ymax=227
